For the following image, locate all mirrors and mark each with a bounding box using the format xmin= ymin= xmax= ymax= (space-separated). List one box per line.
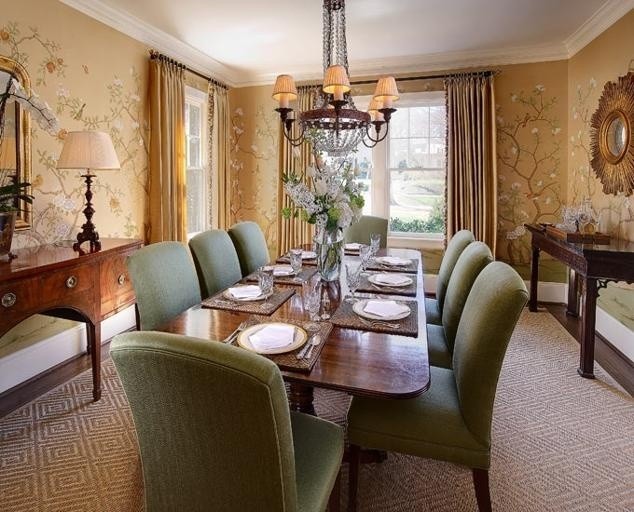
xmin=587 ymin=70 xmax=633 ymax=199
xmin=0 ymin=55 xmax=36 ymax=234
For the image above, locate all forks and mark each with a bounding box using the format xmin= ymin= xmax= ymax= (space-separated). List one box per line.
xmin=222 ymin=320 xmax=248 ymax=344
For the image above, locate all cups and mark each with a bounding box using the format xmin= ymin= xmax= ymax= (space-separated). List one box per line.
xmin=555 ymin=197 xmax=599 ymax=234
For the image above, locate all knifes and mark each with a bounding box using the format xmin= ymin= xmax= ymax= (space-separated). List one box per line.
xmin=296 ymin=332 xmax=321 ymax=360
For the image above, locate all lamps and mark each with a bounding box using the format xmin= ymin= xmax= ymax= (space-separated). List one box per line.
xmin=54 ymin=128 xmax=121 ymax=253
xmin=267 ymin=1 xmax=403 ymax=161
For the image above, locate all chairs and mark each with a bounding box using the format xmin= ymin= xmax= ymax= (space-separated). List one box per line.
xmin=342 ymin=214 xmax=389 ymax=249
xmin=424 ymin=228 xmax=477 ymax=325
xmin=425 ymin=240 xmax=496 ymax=369
xmin=343 ymin=259 xmax=532 ymax=512
xmin=225 ymin=219 xmax=270 ymax=278
xmin=106 ymin=328 xmax=347 ymax=511
xmin=124 ymin=240 xmax=205 ymax=335
xmin=187 ymin=227 xmax=244 ymax=300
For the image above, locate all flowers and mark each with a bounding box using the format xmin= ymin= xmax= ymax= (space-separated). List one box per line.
xmin=277 ymin=124 xmax=371 ymax=282
xmin=0 ymin=55 xmax=62 ymax=215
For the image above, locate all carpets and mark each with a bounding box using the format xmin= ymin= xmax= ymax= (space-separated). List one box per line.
xmin=1 ymin=301 xmax=634 ymax=512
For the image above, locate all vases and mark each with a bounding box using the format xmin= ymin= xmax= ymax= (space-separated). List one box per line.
xmin=0 ymin=211 xmax=20 ymax=261
xmin=313 ymin=220 xmax=347 ymax=281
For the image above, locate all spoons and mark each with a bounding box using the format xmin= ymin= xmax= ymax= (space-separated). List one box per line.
xmin=305 ymin=333 xmax=320 ymax=360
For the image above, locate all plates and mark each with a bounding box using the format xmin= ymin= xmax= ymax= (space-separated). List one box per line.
xmin=539 ymin=222 xmax=554 ymax=227
xmin=222 ymin=233 xmax=414 ymax=328
xmin=235 ymin=322 xmax=308 ymax=355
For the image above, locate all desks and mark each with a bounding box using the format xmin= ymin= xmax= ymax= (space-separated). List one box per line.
xmin=1 ymin=233 xmax=145 ymax=403
xmin=520 ymin=219 xmax=634 ymax=381
